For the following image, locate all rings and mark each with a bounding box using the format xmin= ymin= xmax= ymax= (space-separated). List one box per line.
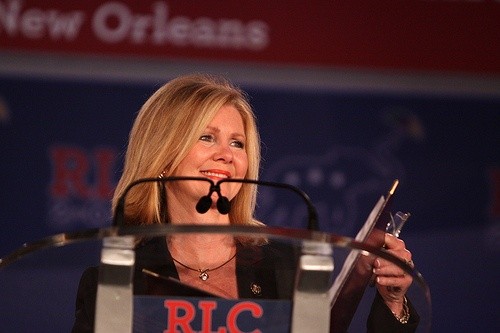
xmin=408 ymin=260 xmax=411 ymax=267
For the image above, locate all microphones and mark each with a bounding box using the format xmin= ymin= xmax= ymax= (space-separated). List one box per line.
xmin=113 ymin=175 xmax=214 ymax=226
xmin=216 ymin=178 xmax=319 ymax=232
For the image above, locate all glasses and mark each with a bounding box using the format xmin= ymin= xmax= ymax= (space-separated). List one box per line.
xmin=383 ymin=210 xmax=411 ymax=249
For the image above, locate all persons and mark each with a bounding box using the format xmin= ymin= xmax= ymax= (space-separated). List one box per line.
xmin=71 ymin=72 xmax=421 ymax=333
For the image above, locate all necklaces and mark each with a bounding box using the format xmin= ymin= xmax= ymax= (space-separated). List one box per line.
xmin=172 ymin=252 xmax=238 ymax=281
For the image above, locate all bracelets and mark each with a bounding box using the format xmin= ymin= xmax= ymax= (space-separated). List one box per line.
xmin=387 ymin=295 xmax=410 ymax=324
